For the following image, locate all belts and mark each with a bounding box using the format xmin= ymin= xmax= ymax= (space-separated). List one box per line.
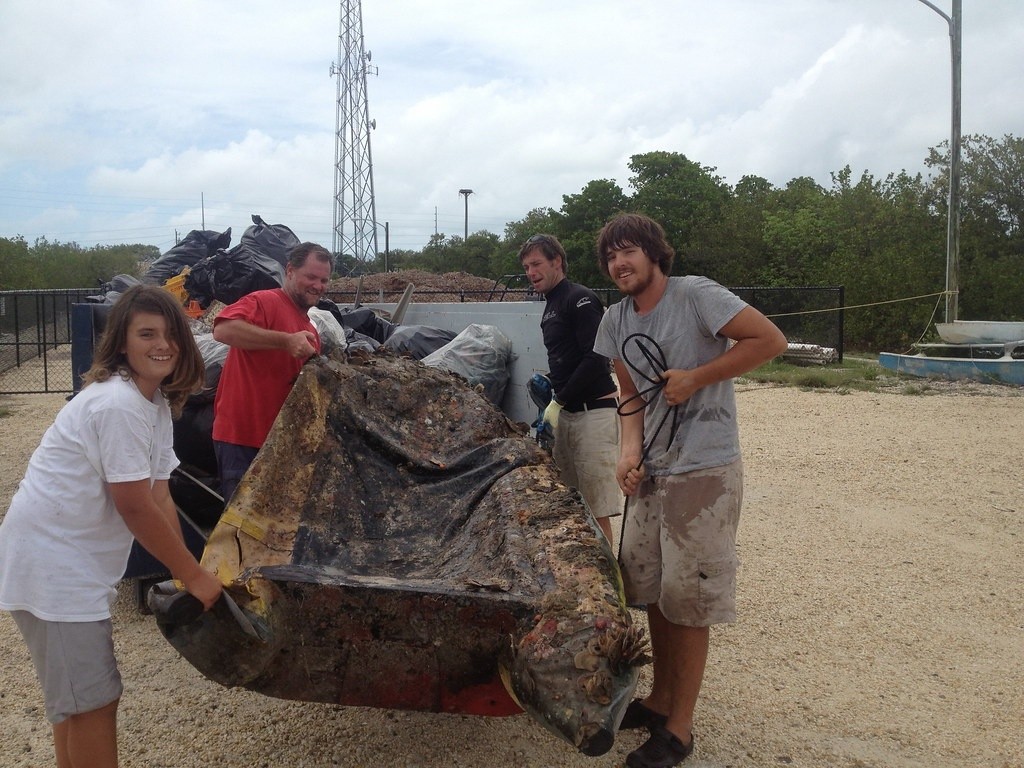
xmin=561 ymin=397 xmax=620 ymax=413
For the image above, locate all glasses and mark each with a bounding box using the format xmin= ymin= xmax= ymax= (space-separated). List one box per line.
xmin=525 ymin=234 xmax=564 ymax=258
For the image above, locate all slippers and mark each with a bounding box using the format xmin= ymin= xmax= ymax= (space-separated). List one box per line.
xmin=625 ymin=730 xmax=694 ymax=768
xmin=616 ymin=697 xmax=669 ymax=730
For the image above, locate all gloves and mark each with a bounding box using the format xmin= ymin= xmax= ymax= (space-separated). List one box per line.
xmin=530 ymin=373 xmax=551 ymax=396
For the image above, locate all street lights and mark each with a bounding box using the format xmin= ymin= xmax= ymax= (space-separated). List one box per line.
xmin=352 ymin=218 xmax=388 ymax=274
xmin=459 ymin=188 xmax=474 ymax=242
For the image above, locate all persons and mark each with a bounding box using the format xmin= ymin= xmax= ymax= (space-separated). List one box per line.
xmin=212 ymin=242 xmax=332 ymax=508
xmin=593 ymin=214 xmax=787 ymax=768
xmin=515 ymin=234 xmax=622 ymax=549
xmin=1 ymin=284 xmax=223 ymax=768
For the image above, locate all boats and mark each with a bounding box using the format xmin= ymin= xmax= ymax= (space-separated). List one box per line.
xmin=935 ymin=320 xmax=1024 ymax=344
xmin=880 ymin=352 xmax=1024 ymax=386
